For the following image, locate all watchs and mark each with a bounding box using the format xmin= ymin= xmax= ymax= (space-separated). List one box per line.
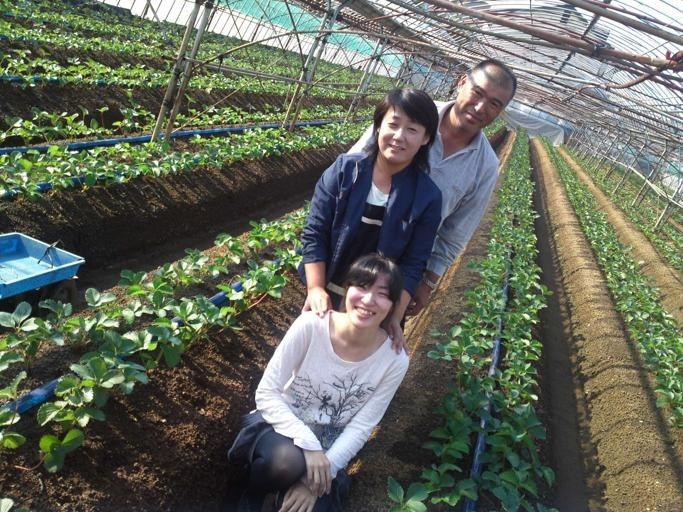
xmin=421 ymin=276 xmax=442 ymax=292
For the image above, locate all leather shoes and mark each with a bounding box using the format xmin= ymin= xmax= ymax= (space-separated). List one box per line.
xmin=236 ymin=491 xmax=265 ymax=509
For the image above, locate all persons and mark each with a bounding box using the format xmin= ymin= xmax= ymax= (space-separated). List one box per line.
xmin=227 ymin=255 xmax=408 ymax=512
xmin=297 ymin=88 xmax=442 ymax=354
xmin=345 ymin=59 xmax=517 ymax=316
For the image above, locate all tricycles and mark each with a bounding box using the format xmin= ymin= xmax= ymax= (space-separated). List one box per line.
xmin=0 ymin=230 xmax=86 ymax=320
